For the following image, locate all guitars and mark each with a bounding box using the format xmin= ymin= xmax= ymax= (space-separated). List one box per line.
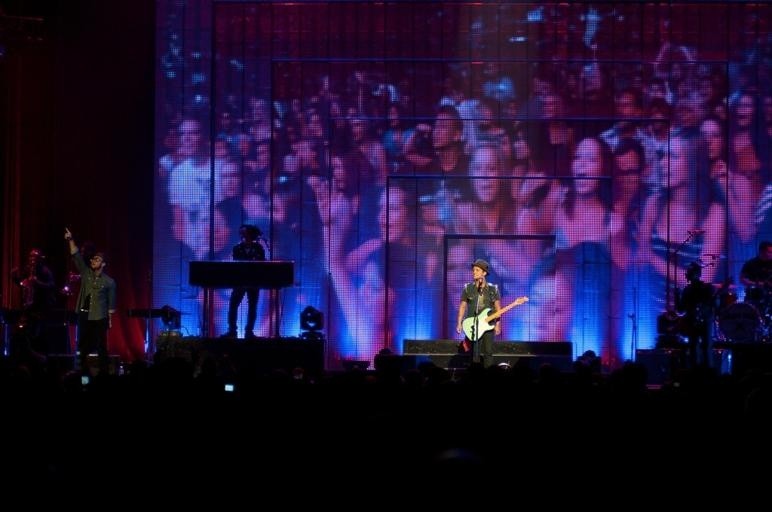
xmin=462 ymin=295 xmax=529 ymax=341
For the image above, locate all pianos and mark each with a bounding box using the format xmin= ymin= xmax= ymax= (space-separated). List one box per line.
xmin=189 ymin=259 xmax=295 ymax=290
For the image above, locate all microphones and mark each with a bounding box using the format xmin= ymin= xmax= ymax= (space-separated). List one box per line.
xmin=478 ymin=279 xmax=481 ymax=292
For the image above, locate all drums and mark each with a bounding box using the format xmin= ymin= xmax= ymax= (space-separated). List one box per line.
xmin=718 ymin=302 xmax=762 ymax=344
xmin=715 ymin=291 xmax=738 ymax=321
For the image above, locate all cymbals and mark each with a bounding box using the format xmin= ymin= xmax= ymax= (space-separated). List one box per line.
xmin=702 ymin=254 xmax=725 ymax=259
xmin=684 ymin=262 xmax=715 ymax=268
xmin=713 ymin=283 xmax=736 ymax=288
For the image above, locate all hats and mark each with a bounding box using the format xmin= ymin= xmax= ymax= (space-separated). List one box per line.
xmin=469 ymin=258 xmax=490 ymax=276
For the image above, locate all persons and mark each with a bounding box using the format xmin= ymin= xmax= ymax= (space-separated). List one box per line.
xmin=0 ymin=225 xmax=772 ymax=512
xmin=153 ymin=0 xmax=772 ymax=370
